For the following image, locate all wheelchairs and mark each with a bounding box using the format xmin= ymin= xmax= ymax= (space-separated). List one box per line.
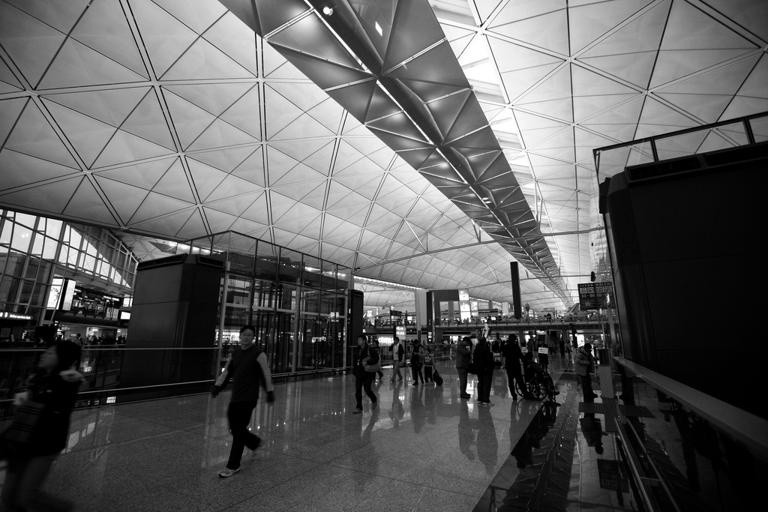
xmin=515 ymin=363 xmax=556 ymax=402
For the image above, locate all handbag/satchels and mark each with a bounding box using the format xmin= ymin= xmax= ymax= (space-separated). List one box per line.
xmin=362 ymin=354 xmax=381 ymax=373
xmin=468 ymin=363 xmax=476 ymax=373
xmin=432 ymin=370 xmax=442 ymax=386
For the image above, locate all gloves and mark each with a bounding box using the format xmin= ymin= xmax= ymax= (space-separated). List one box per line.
xmin=266 ymin=391 xmax=274 ymax=404
xmin=209 ymin=384 xmax=220 ymax=398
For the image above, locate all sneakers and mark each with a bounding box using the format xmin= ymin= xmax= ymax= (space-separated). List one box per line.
xmin=481 ymin=401 xmax=495 ymax=405
xmin=219 ymin=467 xmax=241 ymax=478
xmin=461 ymin=392 xmax=470 ymax=399
xmin=353 ymin=407 xmax=362 ymax=414
xmin=372 ymin=401 xmax=377 ymax=408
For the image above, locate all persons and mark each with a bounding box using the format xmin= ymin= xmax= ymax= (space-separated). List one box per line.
xmin=578 ymin=395 xmax=603 ymax=454
xmin=278 ymin=311 xmax=608 ymax=412
xmin=23 ymin=332 xmax=127 ymax=371
xmin=348 ymin=381 xmax=556 ymax=490
xmin=208 ymin=325 xmax=275 ymax=478
xmin=0 ymin=339 xmax=85 ymax=512
